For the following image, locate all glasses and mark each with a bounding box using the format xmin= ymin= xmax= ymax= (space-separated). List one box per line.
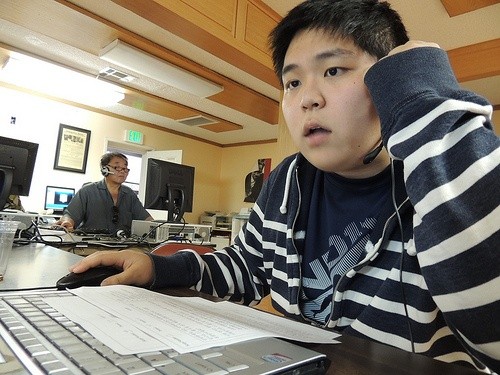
xmin=107 ymin=164 xmax=130 ymax=173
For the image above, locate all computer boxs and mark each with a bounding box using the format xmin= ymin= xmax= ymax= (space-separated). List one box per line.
xmin=129 ymin=219 xmax=213 ymax=243
xmin=0 ymin=212 xmax=38 ymax=237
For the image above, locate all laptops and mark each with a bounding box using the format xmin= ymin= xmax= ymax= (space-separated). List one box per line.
xmin=0 ymin=283 xmax=331 ymax=375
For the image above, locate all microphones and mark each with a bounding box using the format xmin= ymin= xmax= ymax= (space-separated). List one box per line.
xmin=362 ymin=142 xmax=384 ymax=165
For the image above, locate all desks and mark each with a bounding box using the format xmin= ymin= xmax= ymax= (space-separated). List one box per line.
xmin=0 ymin=230 xmax=489 ymax=375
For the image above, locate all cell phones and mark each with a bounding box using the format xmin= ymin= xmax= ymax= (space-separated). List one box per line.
xmin=56 ymin=264 xmax=123 ymax=291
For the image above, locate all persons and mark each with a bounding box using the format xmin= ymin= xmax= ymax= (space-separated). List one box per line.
xmin=51 ymin=152 xmax=154 ymax=232
xmin=69 ymin=0 xmax=500 ymax=375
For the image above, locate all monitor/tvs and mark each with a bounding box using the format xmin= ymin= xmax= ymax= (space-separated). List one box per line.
xmin=142 ymin=157 xmax=196 ymax=224
xmin=0 ymin=137 xmax=39 ymax=213
xmin=44 ymin=186 xmax=77 ymax=215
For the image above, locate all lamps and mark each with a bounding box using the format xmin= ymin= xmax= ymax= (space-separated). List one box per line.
xmin=0 ymin=56 xmax=127 ymax=111
xmin=97 ymin=37 xmax=225 ymax=100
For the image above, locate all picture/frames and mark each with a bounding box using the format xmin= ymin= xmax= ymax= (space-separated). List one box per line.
xmin=53 ymin=123 xmax=92 ymax=174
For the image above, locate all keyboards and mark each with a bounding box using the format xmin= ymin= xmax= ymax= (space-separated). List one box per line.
xmin=67 ymin=227 xmax=129 ymax=234
